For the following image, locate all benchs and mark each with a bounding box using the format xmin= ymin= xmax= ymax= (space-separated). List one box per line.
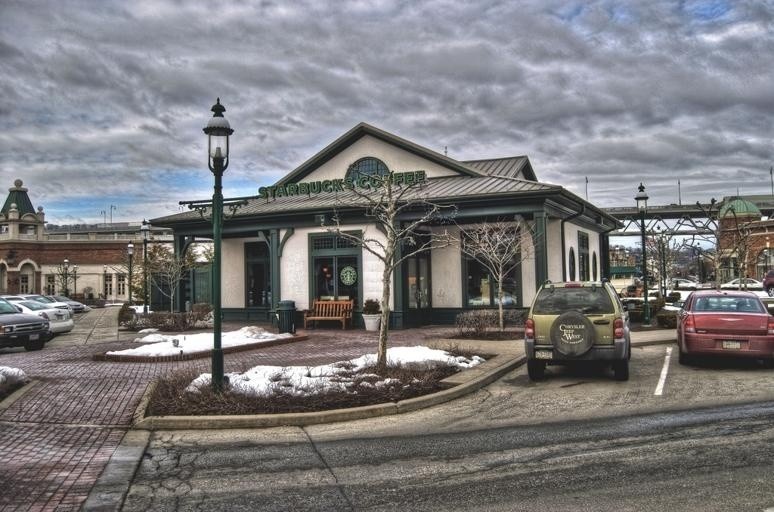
xmin=303 ymin=298 xmax=354 ymax=329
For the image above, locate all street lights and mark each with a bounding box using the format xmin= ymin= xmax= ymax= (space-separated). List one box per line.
xmin=201 ymin=97 xmax=235 ymax=394
xmin=624 ymin=249 xmax=630 ymax=265
xmin=615 ymin=245 xmax=620 ymax=265
xmin=677 ymin=179 xmax=681 ymax=206
xmin=103 ymin=263 xmax=107 ymax=301
xmin=140 ymin=218 xmax=150 ymax=315
xmin=634 ymin=182 xmax=654 ymax=323
xmin=654 ymin=226 xmax=667 ymax=306
xmin=100 ymin=210 xmax=106 ymax=223
xmin=585 ymin=175 xmax=589 ymax=200
xmin=63 ymin=256 xmax=69 ymax=296
xmin=126 ymin=241 xmax=134 ymax=305
xmin=110 ymin=205 xmax=116 ymax=222
xmin=697 ymin=242 xmax=704 ymax=283
xmin=71 ymin=264 xmax=78 ymax=296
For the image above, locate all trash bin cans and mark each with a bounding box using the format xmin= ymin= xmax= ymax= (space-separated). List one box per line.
xmin=275 ymin=300 xmax=297 ymax=334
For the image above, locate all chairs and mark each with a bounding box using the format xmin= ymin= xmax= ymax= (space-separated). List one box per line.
xmin=697 ymin=300 xmax=757 ymax=311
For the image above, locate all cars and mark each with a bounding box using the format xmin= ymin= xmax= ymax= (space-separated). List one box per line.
xmin=637 ymin=275 xmax=655 ymax=284
xmin=653 ymin=278 xmax=702 ymax=290
xmin=677 ymin=288 xmax=773 ymax=366
xmin=720 ymin=277 xmax=764 ymax=289
xmin=0 ymin=293 xmax=83 ymax=351
xmin=609 ymin=276 xmax=641 ymax=295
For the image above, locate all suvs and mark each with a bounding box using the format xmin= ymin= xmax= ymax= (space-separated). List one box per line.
xmin=466 ymin=268 xmax=516 ymax=298
xmin=523 ymin=277 xmax=631 ymax=382
xmin=762 ymin=269 xmax=774 ymax=297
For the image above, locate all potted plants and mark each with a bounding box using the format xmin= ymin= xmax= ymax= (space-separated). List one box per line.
xmin=362 ymin=299 xmax=382 ymax=331
